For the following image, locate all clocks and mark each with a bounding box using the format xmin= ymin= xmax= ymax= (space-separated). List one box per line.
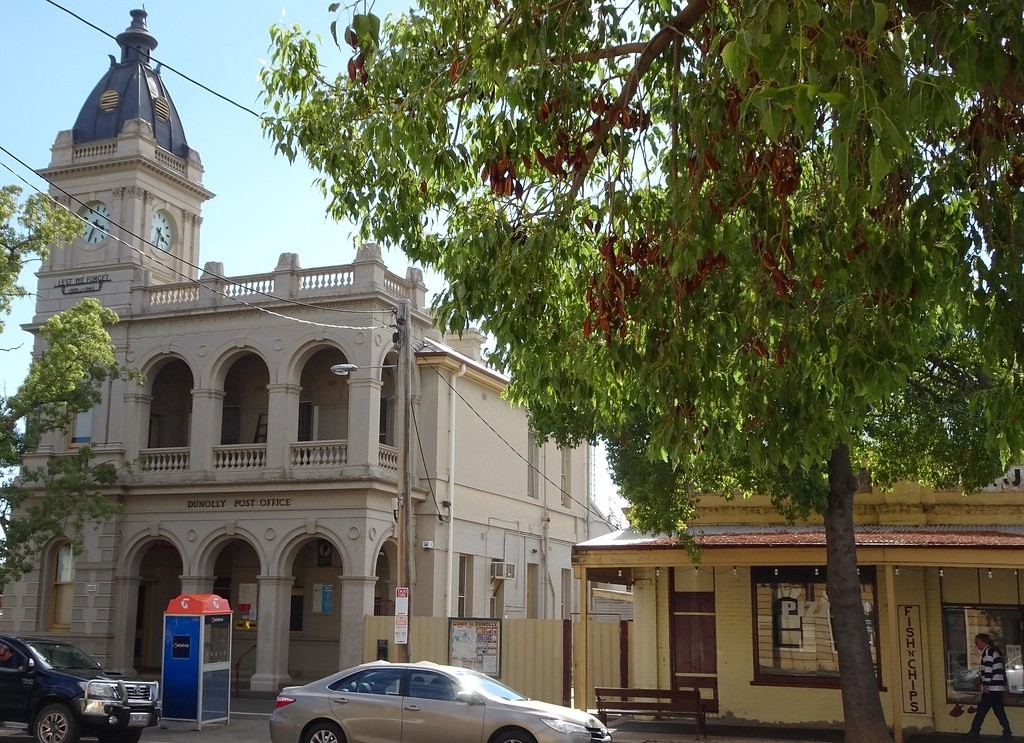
xmin=79 ymin=204 xmax=110 ymax=243
xmin=150 ymin=210 xmax=173 ymax=253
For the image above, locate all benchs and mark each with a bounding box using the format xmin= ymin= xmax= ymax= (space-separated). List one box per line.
xmin=594 ymin=687 xmax=707 ymax=741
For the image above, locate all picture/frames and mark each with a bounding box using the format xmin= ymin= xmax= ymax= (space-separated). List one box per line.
xmin=448 ymin=617 xmax=502 ymax=679
xmin=309 ymin=581 xmax=335 ymax=616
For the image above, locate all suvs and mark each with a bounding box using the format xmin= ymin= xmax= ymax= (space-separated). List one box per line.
xmin=0 ymin=633 xmax=163 ymax=743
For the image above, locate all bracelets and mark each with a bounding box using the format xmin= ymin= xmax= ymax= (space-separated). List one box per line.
xmin=984 ymin=686 xmax=988 ymax=688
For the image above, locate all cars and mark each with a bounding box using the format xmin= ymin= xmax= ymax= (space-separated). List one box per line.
xmin=996 ymin=654 xmax=1024 ymax=693
xmin=268 ymin=660 xmax=613 ymax=743
xmin=952 ymin=669 xmax=981 ymax=691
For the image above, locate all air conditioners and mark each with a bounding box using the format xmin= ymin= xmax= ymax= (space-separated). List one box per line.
xmin=491 ymin=562 xmax=517 ymax=581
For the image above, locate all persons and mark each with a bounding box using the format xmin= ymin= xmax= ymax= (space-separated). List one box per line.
xmin=0 ymin=644 xmax=22 ymax=668
xmin=963 ymin=633 xmax=1012 ymax=739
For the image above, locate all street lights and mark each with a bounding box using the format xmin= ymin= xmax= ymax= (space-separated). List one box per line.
xmin=329 ymin=295 xmax=421 ymax=662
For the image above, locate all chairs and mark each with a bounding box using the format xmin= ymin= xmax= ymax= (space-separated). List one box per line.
xmin=348 ymin=675 xmax=447 ymax=699
